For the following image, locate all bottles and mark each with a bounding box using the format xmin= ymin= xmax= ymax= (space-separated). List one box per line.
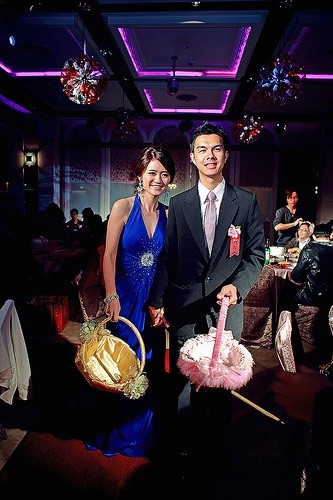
xmin=265 ymin=239 xmax=270 ymax=265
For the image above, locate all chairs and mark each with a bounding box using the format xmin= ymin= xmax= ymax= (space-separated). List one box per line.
xmin=69 ymin=243 xmax=106 ymax=323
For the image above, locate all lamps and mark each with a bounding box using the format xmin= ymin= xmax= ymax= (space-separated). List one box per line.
xmin=166 ymin=56 xmax=180 ymax=95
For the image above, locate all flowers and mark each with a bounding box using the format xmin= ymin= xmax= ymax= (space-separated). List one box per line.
xmin=227 ymin=224 xmax=241 ymax=239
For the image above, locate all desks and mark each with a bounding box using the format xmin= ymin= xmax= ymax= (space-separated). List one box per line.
xmin=242 ymin=268 xmax=291 ymax=341
